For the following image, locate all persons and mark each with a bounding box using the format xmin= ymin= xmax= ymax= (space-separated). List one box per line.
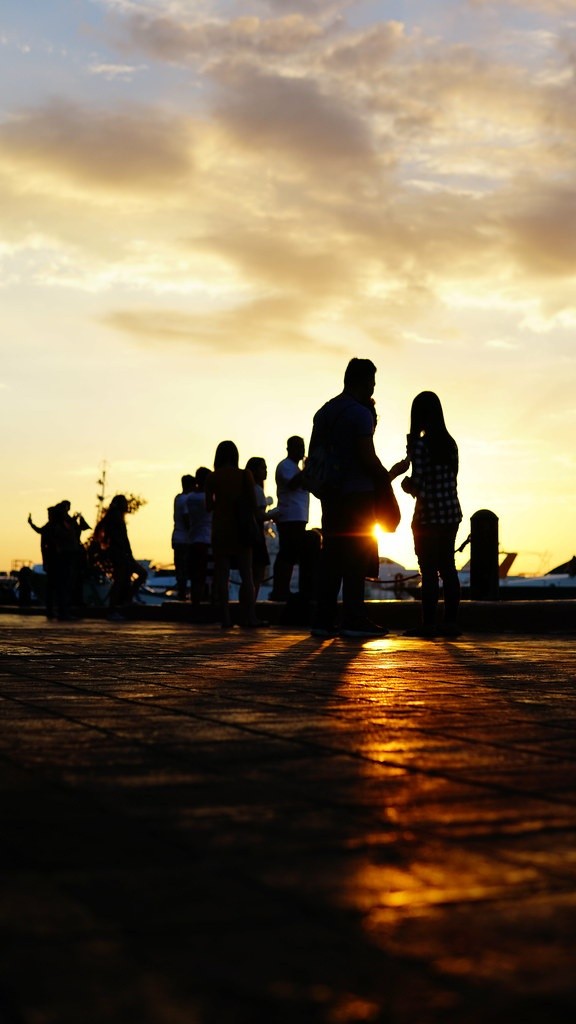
xmin=403 ymin=391 xmax=463 ymax=640
xmin=173 ymin=356 xmax=408 ymax=640
xmin=26 ymin=495 xmax=149 ymax=622
